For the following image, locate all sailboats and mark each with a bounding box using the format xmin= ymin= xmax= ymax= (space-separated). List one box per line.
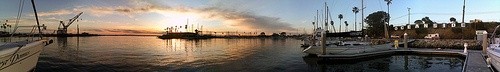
xmin=0 ymin=0 xmax=47 ymax=72
xmin=303 ymin=0 xmax=394 ymax=59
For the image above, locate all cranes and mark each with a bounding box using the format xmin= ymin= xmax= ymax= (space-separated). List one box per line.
xmin=57 ymin=10 xmax=83 ymax=35
